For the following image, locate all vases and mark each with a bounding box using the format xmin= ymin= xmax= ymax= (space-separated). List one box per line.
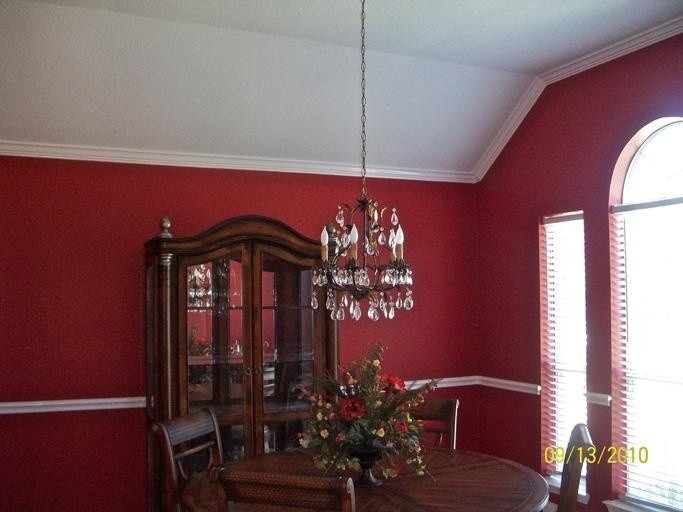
xmin=289 ymin=342 xmax=445 ymax=486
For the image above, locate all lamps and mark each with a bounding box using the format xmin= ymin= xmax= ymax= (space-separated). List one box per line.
xmin=556 ymin=424 xmax=596 ymax=511
xmin=148 ymin=407 xmax=226 ymax=511
xmin=209 ymin=468 xmax=357 ymax=511
xmin=401 ymin=396 xmax=461 ymax=451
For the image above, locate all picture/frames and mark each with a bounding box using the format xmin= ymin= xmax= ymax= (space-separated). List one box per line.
xmin=181 ymin=444 xmax=550 ymax=512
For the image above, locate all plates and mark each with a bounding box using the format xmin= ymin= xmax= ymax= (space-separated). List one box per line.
xmin=141 ymin=213 xmax=341 ymax=511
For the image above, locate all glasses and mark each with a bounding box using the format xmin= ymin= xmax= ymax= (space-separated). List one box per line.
xmin=186 ymin=263 xmax=217 ymax=313
xmin=311 ymin=0 xmax=413 ymax=324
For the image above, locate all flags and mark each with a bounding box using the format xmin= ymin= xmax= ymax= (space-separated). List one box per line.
xmin=349 ymin=448 xmax=387 ymax=486
